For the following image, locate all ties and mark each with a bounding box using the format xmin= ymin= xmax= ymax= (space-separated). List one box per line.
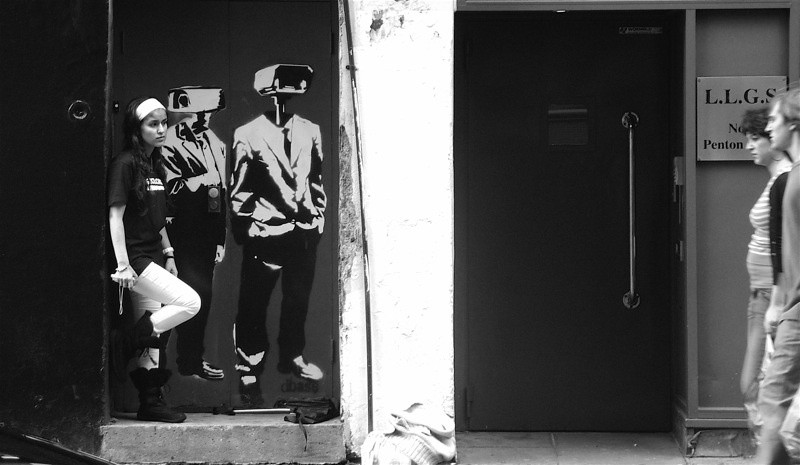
xmin=281 ymin=128 xmax=290 ymax=162
xmin=196 ymin=134 xmax=219 ymax=185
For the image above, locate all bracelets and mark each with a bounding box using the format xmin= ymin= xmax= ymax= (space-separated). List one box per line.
xmin=166 ymin=256 xmax=174 ymax=258
xmin=116 ymin=263 xmax=130 ymax=273
xmin=162 ymin=246 xmax=174 ymax=254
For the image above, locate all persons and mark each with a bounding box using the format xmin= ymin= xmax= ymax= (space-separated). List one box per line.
xmin=106 ymin=98 xmax=202 ymax=424
xmin=740 ymin=86 xmax=800 ymax=465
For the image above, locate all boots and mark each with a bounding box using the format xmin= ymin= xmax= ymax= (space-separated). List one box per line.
xmin=129 ymin=367 xmax=187 ymax=423
xmin=111 ymin=310 xmax=161 ymax=377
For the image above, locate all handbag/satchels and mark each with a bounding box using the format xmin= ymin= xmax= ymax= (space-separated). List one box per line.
xmin=283 ymin=396 xmax=339 ymax=425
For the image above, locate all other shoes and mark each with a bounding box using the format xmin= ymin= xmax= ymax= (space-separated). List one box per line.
xmin=178 ymin=357 xmax=224 ymax=381
xmin=277 ymin=354 xmax=323 ymax=379
xmin=237 ymin=357 xmax=262 ymax=402
